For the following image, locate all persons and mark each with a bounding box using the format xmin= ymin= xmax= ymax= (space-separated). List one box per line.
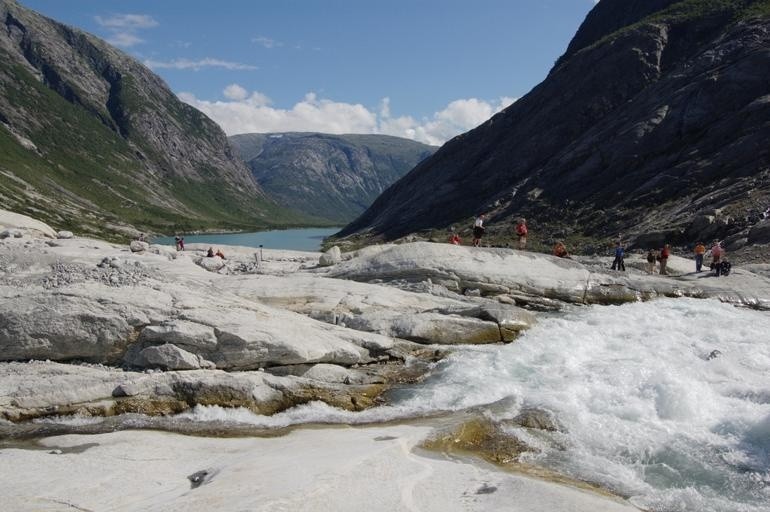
xmin=179 ymin=237 xmax=185 ymax=250
xmin=216 ymin=249 xmax=228 ymax=259
xmin=207 ymin=247 xmax=214 ymax=257
xmin=175 ymin=236 xmax=180 ymax=251
xmin=449 ymin=211 xmax=732 ymax=276
xmin=138 ymin=233 xmax=144 ymax=242
